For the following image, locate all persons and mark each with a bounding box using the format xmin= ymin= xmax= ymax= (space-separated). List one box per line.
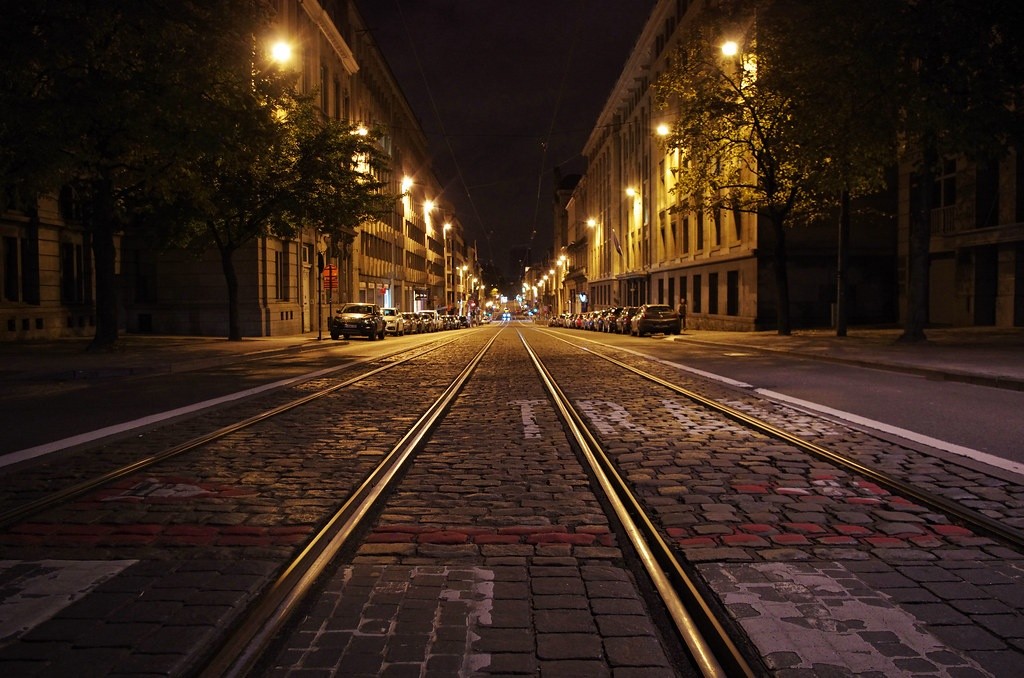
xmin=675 ymin=299 xmax=688 ymax=331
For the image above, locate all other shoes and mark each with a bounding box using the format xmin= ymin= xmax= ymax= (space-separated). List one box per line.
xmin=684 ymin=328 xmax=688 ymax=331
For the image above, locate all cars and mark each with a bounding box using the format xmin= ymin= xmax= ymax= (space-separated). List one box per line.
xmin=379 ymin=307 xmax=405 ymax=336
xmin=615 ymin=306 xmax=640 ymax=334
xmin=400 ymin=304 xmax=493 ymax=335
xmin=330 ymin=301 xmax=386 ymax=341
xmin=630 ymin=304 xmax=684 ymax=336
xmin=547 ymin=304 xmax=623 ymax=333
